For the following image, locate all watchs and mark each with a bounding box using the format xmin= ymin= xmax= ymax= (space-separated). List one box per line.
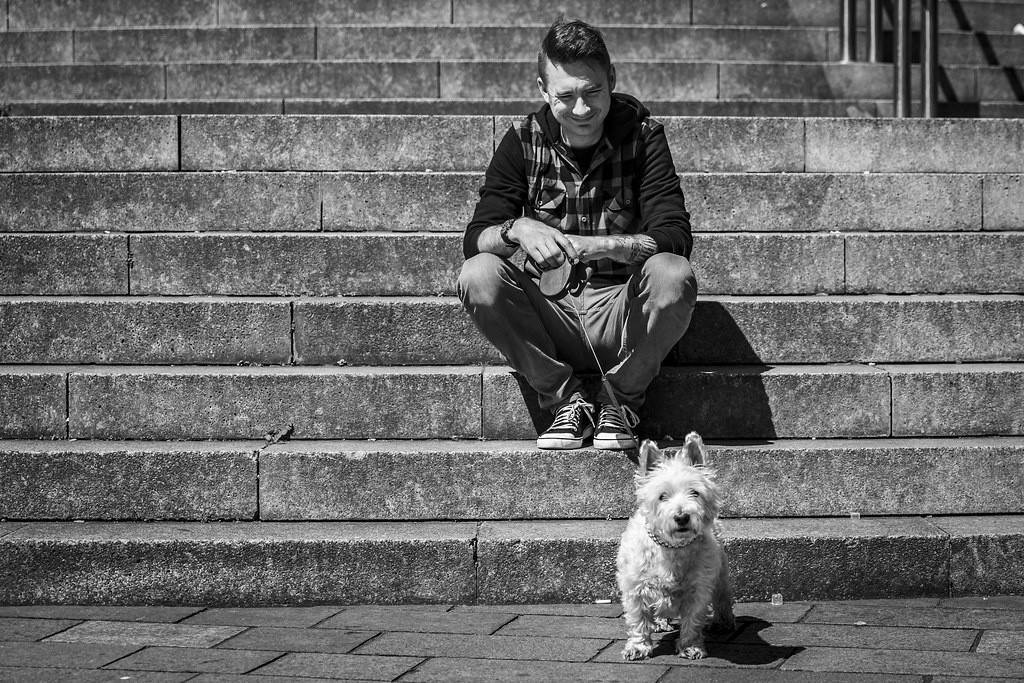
xmin=501 ymin=218 xmax=520 ymax=248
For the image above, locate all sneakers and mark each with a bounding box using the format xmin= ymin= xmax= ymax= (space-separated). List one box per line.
xmin=537 ymin=398 xmax=596 ymax=448
xmin=593 ymin=405 xmax=639 ymax=449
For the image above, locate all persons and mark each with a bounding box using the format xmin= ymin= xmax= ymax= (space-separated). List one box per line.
xmin=456 ymin=17 xmax=698 ymax=449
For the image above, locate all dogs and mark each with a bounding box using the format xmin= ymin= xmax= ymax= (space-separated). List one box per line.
xmin=614 ymin=431 xmax=737 ymax=660
